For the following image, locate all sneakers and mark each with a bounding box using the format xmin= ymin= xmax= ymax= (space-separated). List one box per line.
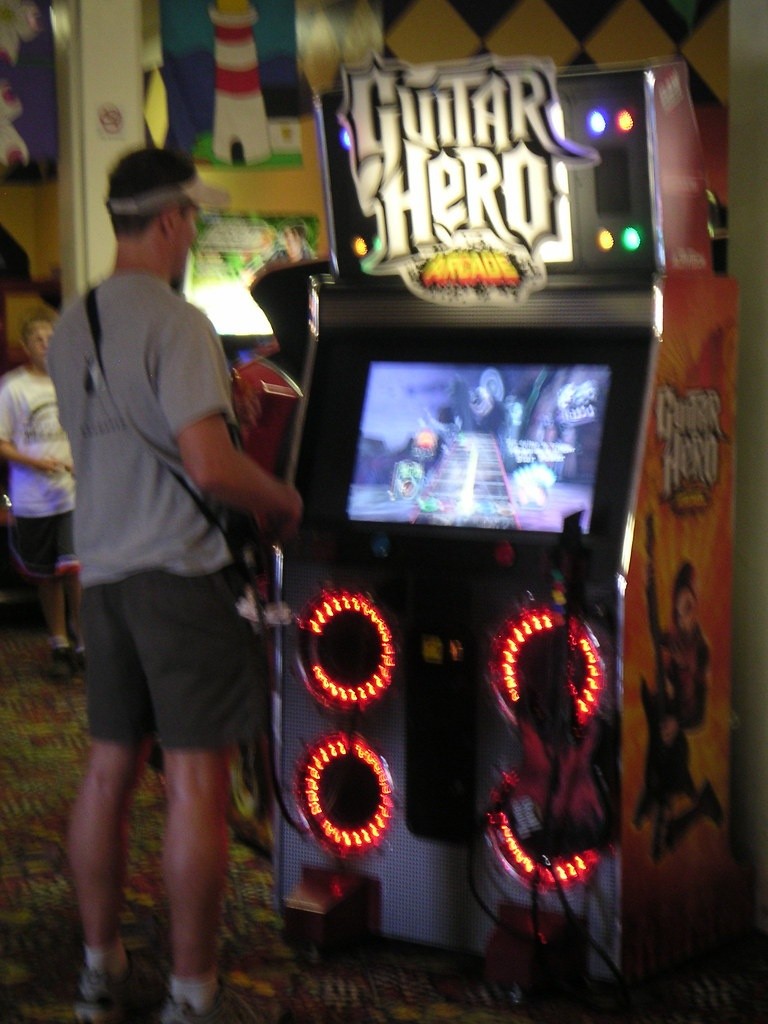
xmin=158 ymin=976 xmax=292 ymax=1024
xmin=74 ymin=950 xmax=166 ymax=1024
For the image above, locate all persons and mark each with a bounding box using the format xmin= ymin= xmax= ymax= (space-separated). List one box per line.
xmin=46 ymin=150 xmax=303 ymax=1023
xmin=232 ymin=263 xmax=312 ymax=602
xmin=0 ymin=315 xmax=84 ymax=683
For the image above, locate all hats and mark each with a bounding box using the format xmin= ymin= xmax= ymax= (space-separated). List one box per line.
xmin=108 ymin=174 xmax=231 ymax=216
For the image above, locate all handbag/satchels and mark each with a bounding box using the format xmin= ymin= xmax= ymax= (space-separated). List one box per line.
xmin=219 ymin=416 xmax=256 ymax=574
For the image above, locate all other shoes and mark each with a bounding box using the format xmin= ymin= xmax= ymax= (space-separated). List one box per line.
xmin=51 ymin=646 xmax=81 ymax=674
xmin=74 ymin=648 xmax=86 ymax=672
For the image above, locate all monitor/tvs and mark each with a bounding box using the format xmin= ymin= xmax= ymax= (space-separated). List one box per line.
xmin=345 ymin=360 xmax=611 ymax=534
